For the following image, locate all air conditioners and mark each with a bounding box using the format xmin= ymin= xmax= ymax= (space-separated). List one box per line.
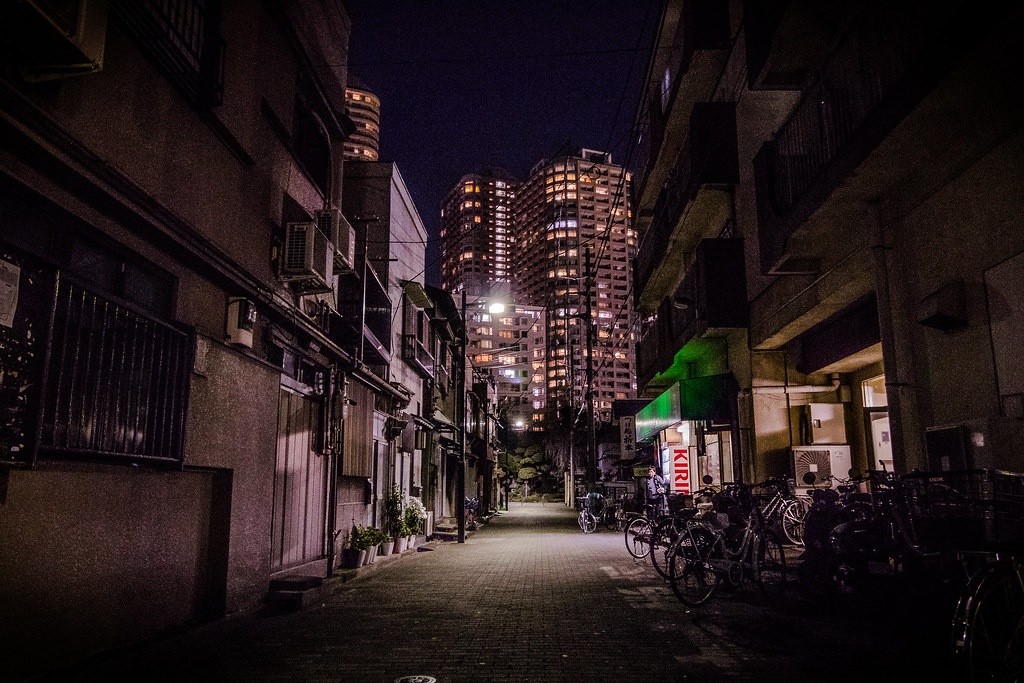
xmin=787 ymin=445 xmax=854 ymax=487
xmin=313 ymin=209 xmax=356 ymax=274
xmin=284 ymin=221 xmax=335 ymax=292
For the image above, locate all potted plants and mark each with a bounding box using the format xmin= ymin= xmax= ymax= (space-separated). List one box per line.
xmin=382 ymin=530 xmax=395 ymax=555
xmin=400 ymin=525 xmax=410 ymax=550
xmin=358 ymin=528 xmax=372 ymax=565
xmin=368 ymin=526 xmax=384 ymax=562
xmin=406 ymin=507 xmax=423 ymax=548
xmin=384 ymin=482 xmax=405 ymax=553
xmin=344 ymin=526 xmax=370 ymax=569
xmin=364 ymin=529 xmax=376 ymax=563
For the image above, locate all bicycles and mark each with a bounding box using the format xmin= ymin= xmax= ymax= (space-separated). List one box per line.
xmin=571 ymin=452 xmax=1024 ymax=683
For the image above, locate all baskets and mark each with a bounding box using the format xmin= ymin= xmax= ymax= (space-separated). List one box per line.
xmin=670 ymin=494 xmax=693 ymax=513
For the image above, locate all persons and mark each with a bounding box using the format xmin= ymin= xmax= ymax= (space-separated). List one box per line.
xmin=645 ymin=466 xmax=665 ymax=520
